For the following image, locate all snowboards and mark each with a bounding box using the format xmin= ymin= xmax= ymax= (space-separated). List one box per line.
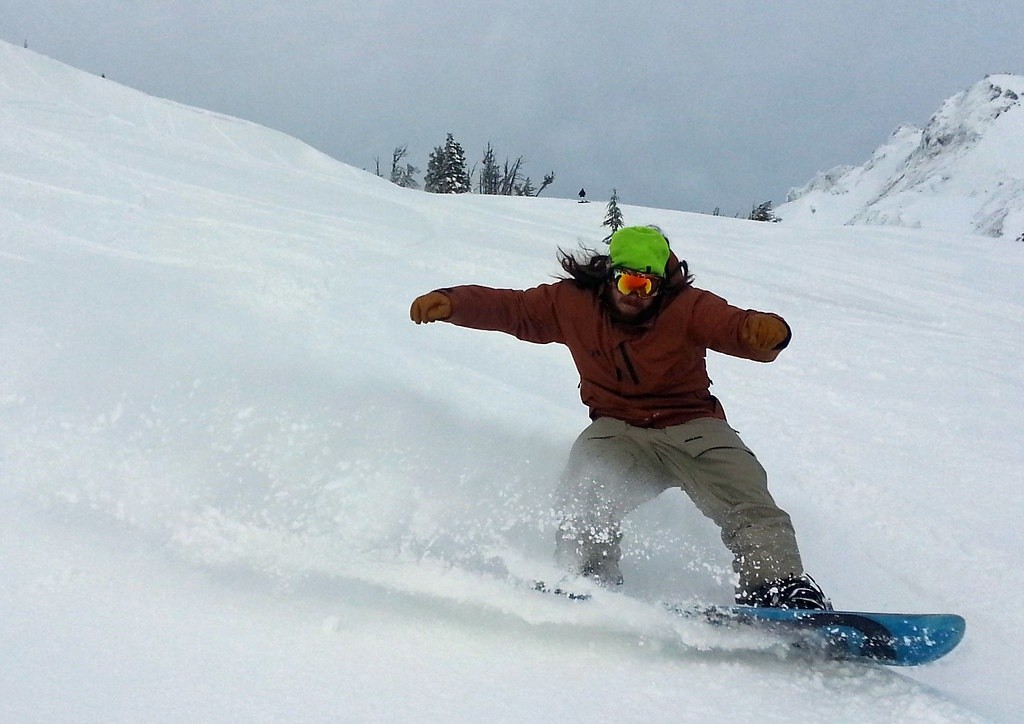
xmin=531 ymin=579 xmax=964 ymax=666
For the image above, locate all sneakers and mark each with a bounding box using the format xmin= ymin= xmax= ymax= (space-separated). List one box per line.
xmin=551 ymin=570 xmax=626 ymax=593
xmin=737 ymin=577 xmax=821 ymax=610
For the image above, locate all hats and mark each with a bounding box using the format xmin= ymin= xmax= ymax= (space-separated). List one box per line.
xmin=608 ymin=226 xmax=681 ymax=281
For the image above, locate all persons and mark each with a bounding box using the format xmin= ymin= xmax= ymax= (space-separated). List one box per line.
xmin=578 ymin=188 xmax=586 ymax=202
xmin=410 ymin=227 xmax=832 ymax=611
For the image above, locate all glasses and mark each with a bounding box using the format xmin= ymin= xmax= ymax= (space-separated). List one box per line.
xmin=610 ymin=266 xmax=668 ymax=299
